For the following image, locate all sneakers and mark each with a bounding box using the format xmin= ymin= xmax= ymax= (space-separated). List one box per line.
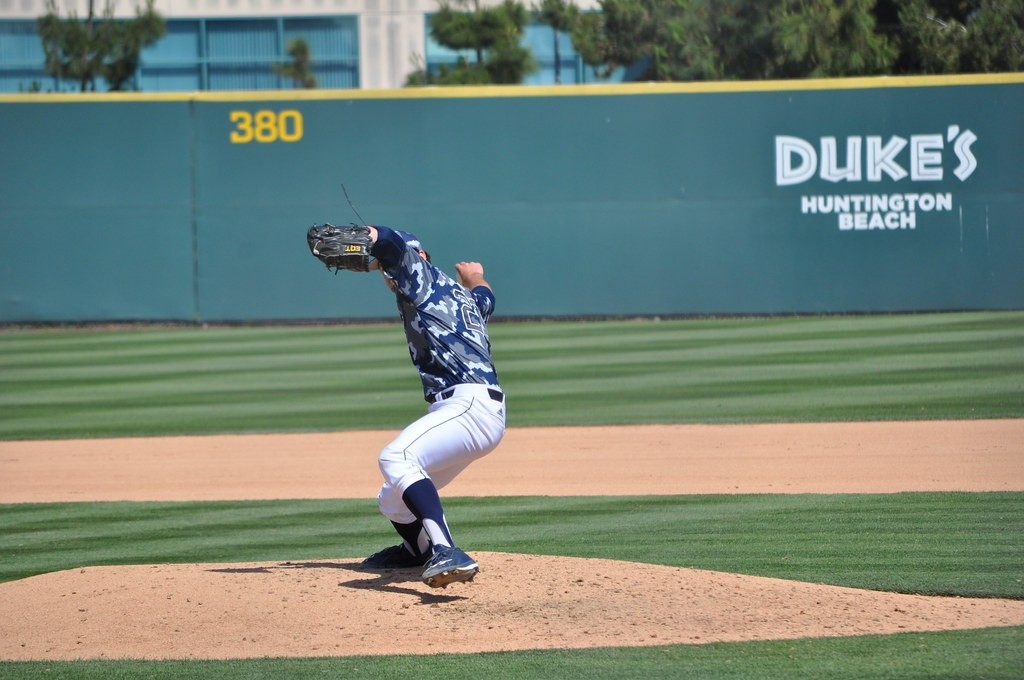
xmin=363 ymin=543 xmax=423 ymax=570
xmin=422 ymin=543 xmax=480 ymax=588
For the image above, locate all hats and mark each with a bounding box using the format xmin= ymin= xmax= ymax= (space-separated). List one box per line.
xmin=399 ymin=230 xmax=423 ymax=250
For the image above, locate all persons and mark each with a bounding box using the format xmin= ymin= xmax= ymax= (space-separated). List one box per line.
xmin=306 ymin=223 xmax=506 ymax=590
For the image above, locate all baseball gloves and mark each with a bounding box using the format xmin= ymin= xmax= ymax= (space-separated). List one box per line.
xmin=306 ymin=223 xmax=372 ymax=273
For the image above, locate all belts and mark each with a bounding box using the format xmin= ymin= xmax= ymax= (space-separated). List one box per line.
xmin=431 ymin=389 xmax=503 ymax=404
